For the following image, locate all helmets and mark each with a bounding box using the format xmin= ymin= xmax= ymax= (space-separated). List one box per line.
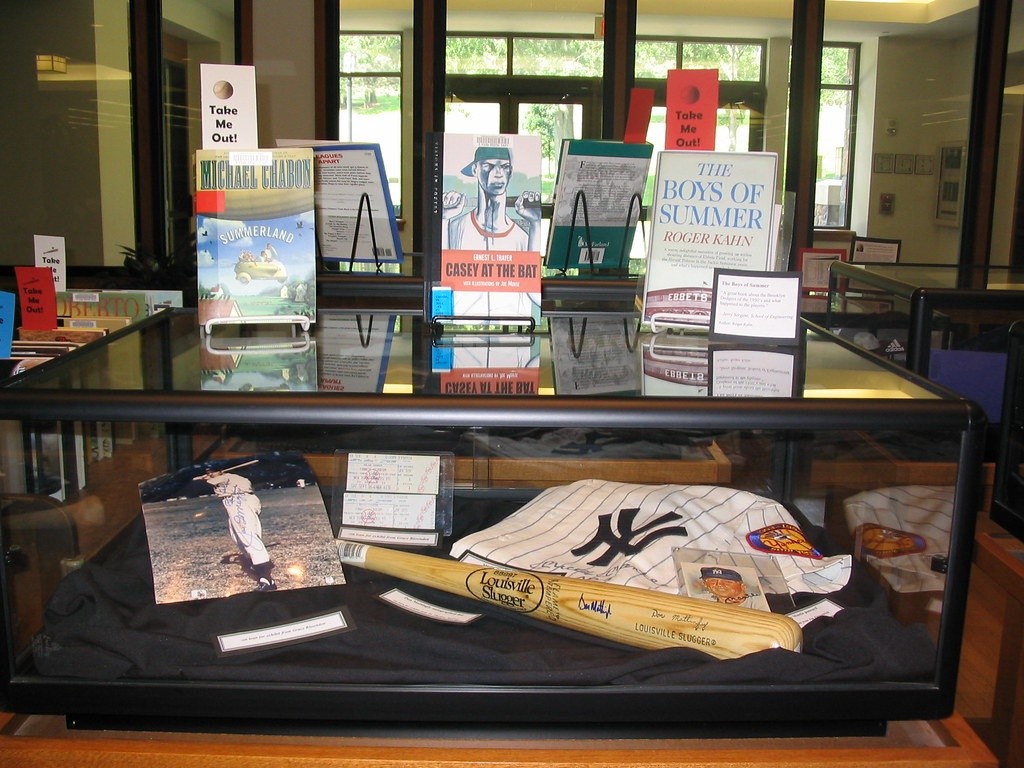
xmin=199 ymin=461 xmax=224 ymax=472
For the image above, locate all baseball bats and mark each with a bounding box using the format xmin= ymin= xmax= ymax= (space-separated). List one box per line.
xmin=192 ymin=459 xmax=259 ymax=481
xmin=333 ymin=541 xmax=804 ymax=661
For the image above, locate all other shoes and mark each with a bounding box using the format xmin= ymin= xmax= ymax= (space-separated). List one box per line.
xmin=256 ymin=575 xmax=279 ymax=594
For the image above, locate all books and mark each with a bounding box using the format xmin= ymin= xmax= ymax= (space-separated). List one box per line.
xmin=274 ymin=136 xmax=403 ymax=264
xmin=199 ymin=337 xmax=318 ymax=392
xmin=0 ymin=289 xmax=183 ymax=502
xmin=316 ymin=313 xmax=398 ymax=394
xmin=543 ymin=136 xmax=655 ymax=270
xmin=194 ymin=148 xmax=317 ymax=324
xmin=421 ymin=133 xmax=541 ymax=327
xmin=548 ymin=315 xmax=642 ymax=399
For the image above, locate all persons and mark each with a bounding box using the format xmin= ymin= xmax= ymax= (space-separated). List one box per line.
xmin=204 ymin=464 xmax=278 ymax=592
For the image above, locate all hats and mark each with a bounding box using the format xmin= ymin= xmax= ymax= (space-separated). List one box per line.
xmin=698 ymin=566 xmax=743 ymax=579
